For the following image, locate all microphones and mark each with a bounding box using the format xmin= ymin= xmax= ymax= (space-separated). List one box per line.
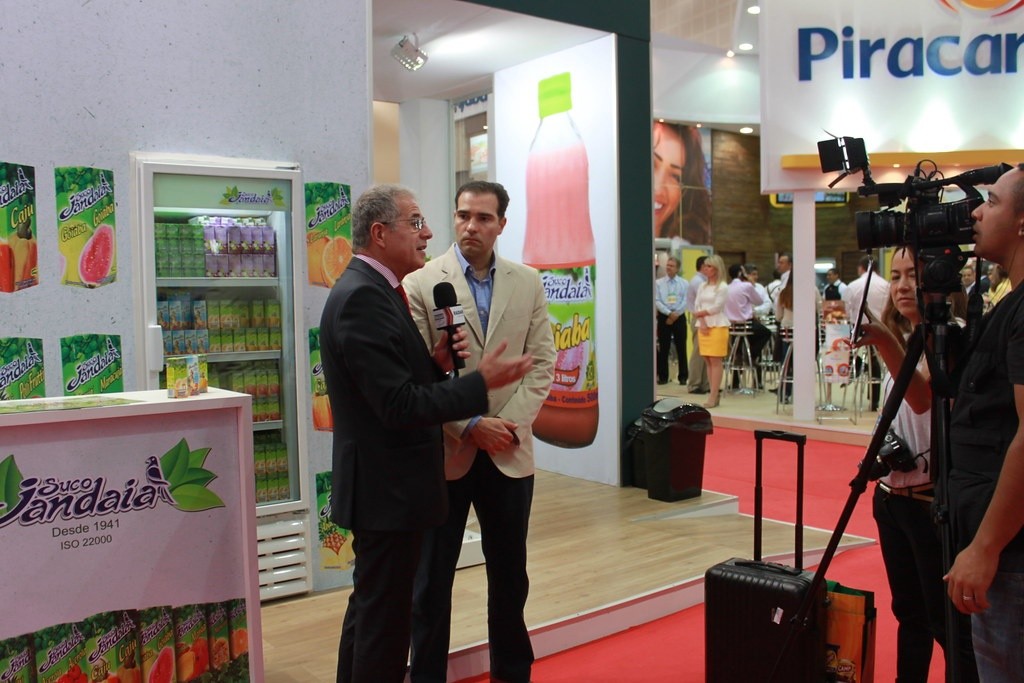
xmin=433 ymin=282 xmax=467 ymax=369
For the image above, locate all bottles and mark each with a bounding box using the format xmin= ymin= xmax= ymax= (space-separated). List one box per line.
xmin=521 ymin=73 xmax=598 ymax=448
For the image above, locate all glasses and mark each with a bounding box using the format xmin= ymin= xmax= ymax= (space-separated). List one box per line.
xmin=377 ymin=216 xmax=428 ymax=232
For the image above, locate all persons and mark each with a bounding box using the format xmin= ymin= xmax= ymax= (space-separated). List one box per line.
xmin=941 ymin=161 xmax=1024 ymax=683
xmin=652 ymin=119 xmax=711 ymax=246
xmin=401 ymin=179 xmax=558 ymax=683
xmin=656 ymin=239 xmax=1018 ymax=409
xmin=318 ymin=185 xmax=535 ymax=682
xmin=849 ymin=245 xmax=976 ymax=683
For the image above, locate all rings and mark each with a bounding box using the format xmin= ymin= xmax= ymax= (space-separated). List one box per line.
xmin=962 ymin=596 xmax=974 ymax=601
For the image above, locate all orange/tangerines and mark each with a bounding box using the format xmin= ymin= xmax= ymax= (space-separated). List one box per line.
xmin=307 ymin=232 xmax=355 ymax=287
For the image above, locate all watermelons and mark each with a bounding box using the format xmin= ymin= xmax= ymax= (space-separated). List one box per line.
xmin=77 ymin=224 xmax=116 ymax=288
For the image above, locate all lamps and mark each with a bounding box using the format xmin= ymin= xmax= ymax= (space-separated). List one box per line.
xmin=817 ymin=136 xmax=877 ymax=189
xmin=391 ymin=32 xmax=428 ymax=72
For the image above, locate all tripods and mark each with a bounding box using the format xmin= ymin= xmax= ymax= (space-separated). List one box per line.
xmin=768 ymin=245 xmax=975 ymax=683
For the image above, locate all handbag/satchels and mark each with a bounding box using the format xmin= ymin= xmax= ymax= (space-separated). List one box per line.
xmin=815 ymin=578 xmax=877 ymax=683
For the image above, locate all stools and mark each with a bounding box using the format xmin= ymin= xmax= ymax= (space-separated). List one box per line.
xmin=722 ymin=321 xmax=886 ymax=425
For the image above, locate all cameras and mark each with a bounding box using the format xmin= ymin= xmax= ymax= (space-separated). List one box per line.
xmin=858 ymin=429 xmax=916 ymax=482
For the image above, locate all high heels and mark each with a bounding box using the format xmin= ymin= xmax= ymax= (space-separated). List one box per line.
xmin=703 ymin=393 xmax=721 ymax=409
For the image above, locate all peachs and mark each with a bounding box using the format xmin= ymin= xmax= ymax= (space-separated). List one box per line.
xmin=148 ymin=637 xmax=210 ymax=683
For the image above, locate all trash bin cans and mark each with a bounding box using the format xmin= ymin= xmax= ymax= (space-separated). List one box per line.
xmin=622 ymin=417 xmax=647 ymax=490
xmin=640 ymin=397 xmax=714 ymax=504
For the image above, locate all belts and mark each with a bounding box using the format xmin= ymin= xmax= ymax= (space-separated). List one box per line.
xmin=876 ymin=480 xmax=935 ymax=502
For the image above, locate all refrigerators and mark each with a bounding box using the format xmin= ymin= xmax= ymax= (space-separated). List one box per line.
xmin=130 ymin=152 xmax=313 ymax=604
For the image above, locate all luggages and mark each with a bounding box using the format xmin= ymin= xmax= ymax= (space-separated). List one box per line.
xmin=705 ymin=429 xmax=824 ymax=683
